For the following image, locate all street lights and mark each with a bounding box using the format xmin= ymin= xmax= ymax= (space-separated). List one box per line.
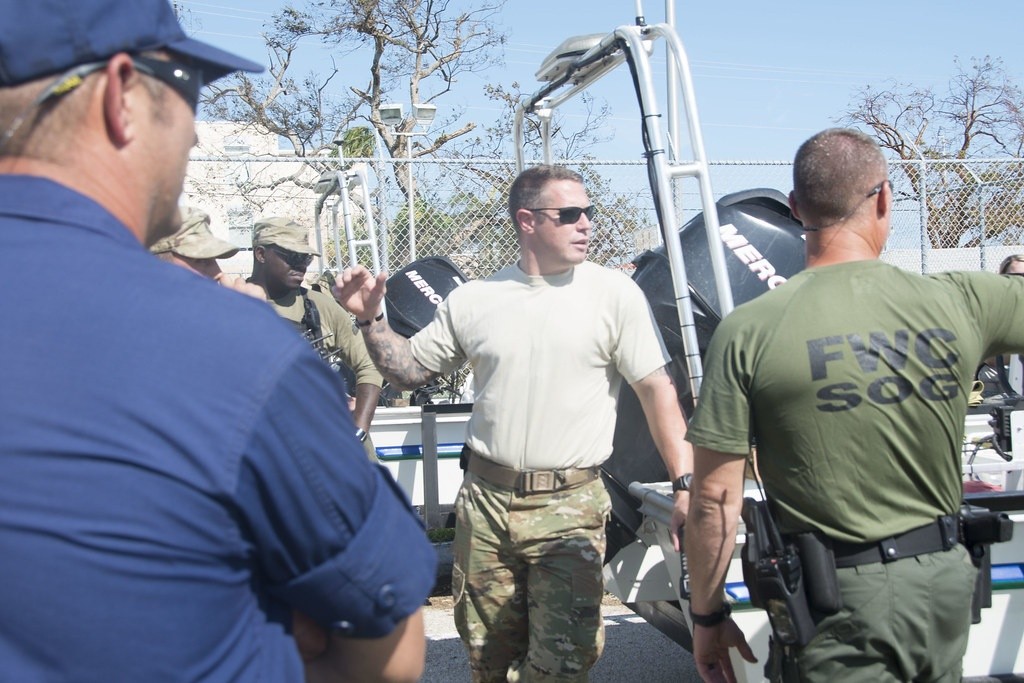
xmin=378 ymin=102 xmax=436 ymax=265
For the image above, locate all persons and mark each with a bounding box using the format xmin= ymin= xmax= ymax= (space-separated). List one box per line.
xmin=977 ymin=253 xmax=1024 ymax=388
xmin=148 ymin=208 xmax=385 ymax=466
xmin=331 ymin=165 xmax=694 ymax=682
xmin=684 ymin=128 xmax=1024 ymax=683
xmin=0 ymin=0 xmax=442 ymax=683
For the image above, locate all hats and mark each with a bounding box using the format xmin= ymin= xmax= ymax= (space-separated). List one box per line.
xmin=255 ymin=218 xmax=321 ymax=258
xmin=150 ymin=206 xmax=239 ymax=260
xmin=0 ymin=0 xmax=264 ymax=90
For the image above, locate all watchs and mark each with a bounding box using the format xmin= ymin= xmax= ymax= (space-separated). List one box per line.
xmin=671 ymin=473 xmax=693 ymax=494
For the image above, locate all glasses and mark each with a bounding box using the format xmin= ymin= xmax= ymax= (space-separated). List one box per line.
xmin=529 ymin=205 xmax=595 ymax=225
xmin=267 ymin=246 xmax=313 ymax=269
xmin=0 ymin=59 xmax=202 ymax=152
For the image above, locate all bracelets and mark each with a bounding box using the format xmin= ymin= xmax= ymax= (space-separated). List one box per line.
xmin=355 ymin=312 xmax=384 ymax=327
xmin=352 ymin=424 xmax=368 ymax=443
xmin=687 ymin=599 xmax=732 ymax=626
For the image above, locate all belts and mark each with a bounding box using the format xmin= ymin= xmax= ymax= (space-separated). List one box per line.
xmin=468 ymin=454 xmax=603 ymax=493
xmin=834 ymin=517 xmax=963 ymax=569
